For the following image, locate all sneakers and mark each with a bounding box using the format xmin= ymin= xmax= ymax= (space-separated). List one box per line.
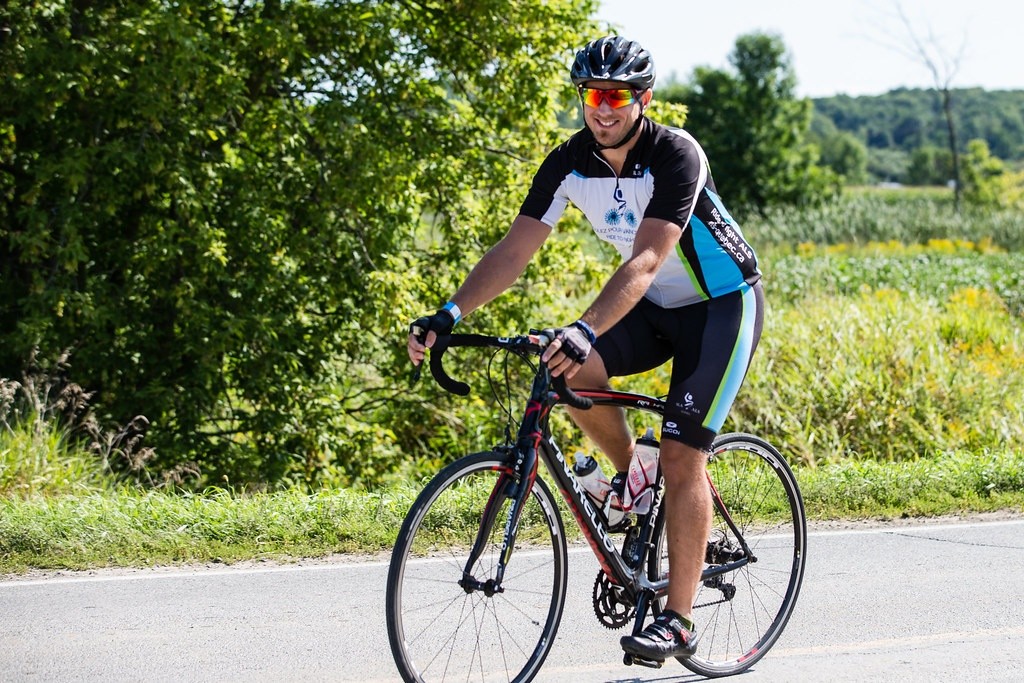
xmin=620 ymin=614 xmax=697 ymax=659
xmin=610 ymin=470 xmax=628 ymax=499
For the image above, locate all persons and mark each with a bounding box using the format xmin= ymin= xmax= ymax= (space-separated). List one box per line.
xmin=407 ymin=37 xmax=764 ymax=660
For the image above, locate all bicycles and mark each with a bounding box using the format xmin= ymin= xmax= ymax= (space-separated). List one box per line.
xmin=385 ymin=316 xmax=808 ymax=683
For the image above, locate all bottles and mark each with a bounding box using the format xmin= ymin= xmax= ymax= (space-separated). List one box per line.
xmin=623 ymin=427 xmax=660 ymax=515
xmin=571 ymin=451 xmax=625 ymax=526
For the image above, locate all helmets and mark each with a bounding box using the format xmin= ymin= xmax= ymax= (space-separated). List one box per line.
xmin=569 ymin=35 xmax=656 ymax=93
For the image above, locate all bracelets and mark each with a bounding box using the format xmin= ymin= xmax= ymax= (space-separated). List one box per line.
xmin=440 ymin=302 xmax=462 ymax=327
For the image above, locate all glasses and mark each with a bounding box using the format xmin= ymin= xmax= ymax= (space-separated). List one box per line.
xmin=579 ymin=86 xmax=650 ymax=109
